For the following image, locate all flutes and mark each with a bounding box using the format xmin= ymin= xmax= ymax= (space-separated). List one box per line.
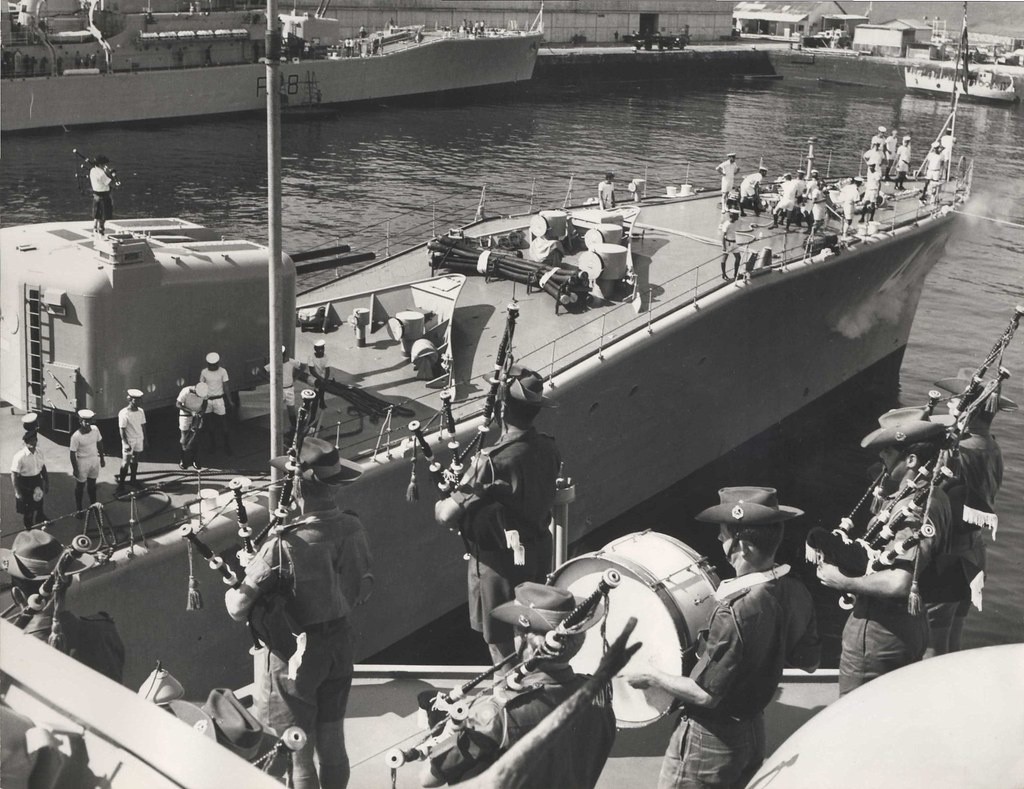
xmin=13 ymin=533 xmax=93 ymax=629
xmin=805 ymin=306 xmax=1024 ymax=612
xmin=179 ymin=388 xmax=317 ymax=591
xmin=409 ymin=301 xmax=520 ymax=560
xmin=245 ymin=618 xmax=308 ymax=750
xmin=382 ymin=564 xmax=620 ymax=771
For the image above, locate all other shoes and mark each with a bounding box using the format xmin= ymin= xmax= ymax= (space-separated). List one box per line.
xmin=192 ymin=460 xmax=200 ymax=469
xmin=179 ymin=458 xmax=186 ymax=468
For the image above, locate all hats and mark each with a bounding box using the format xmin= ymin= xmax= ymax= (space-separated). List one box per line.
xmin=696 ymin=486 xmax=803 ymax=526
xmin=484 ymin=364 xmax=559 ymax=408
xmin=730 ymin=209 xmax=741 ymax=215
xmin=1 ymin=528 xmax=95 ymax=581
xmin=78 ymin=409 xmax=94 ymax=419
xmin=127 ymin=388 xmax=144 ymax=397
xmin=859 ymin=405 xmax=959 ymax=448
xmin=267 ymin=437 xmax=362 ymax=487
xmin=22 ymin=413 xmax=39 ymax=424
xmin=490 ymin=581 xmax=605 ymax=633
xmin=170 ymin=688 xmax=289 ymax=779
xmin=206 ymin=352 xmax=221 ymax=364
xmin=934 ymin=367 xmax=1018 ymax=413
xmin=726 ymin=126 xmax=954 ymax=186
xmin=196 ymin=383 xmax=209 ymax=397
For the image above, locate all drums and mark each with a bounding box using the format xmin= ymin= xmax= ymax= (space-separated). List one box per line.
xmin=544 ymin=525 xmax=724 ymax=731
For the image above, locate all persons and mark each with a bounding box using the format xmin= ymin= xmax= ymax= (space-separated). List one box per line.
xmin=598 ymin=172 xmax=616 ymax=213
xmin=716 ymin=124 xmax=958 ymax=280
xmin=1 ymin=46 xmax=214 ymax=77
xmin=333 ymin=17 xmax=484 ymax=57
xmin=90 ymin=153 xmax=112 ymax=232
xmin=16 ymin=350 xmax=1018 ymax=789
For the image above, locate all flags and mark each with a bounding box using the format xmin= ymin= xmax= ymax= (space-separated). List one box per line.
xmin=959 ymin=22 xmax=970 ymax=95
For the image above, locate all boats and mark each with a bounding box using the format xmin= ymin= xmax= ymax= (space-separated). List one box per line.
xmin=2 ymin=0 xmax=1024 ymax=789
xmin=904 ymin=63 xmax=1015 ymax=102
xmin=1 ymin=0 xmax=544 ymax=132
